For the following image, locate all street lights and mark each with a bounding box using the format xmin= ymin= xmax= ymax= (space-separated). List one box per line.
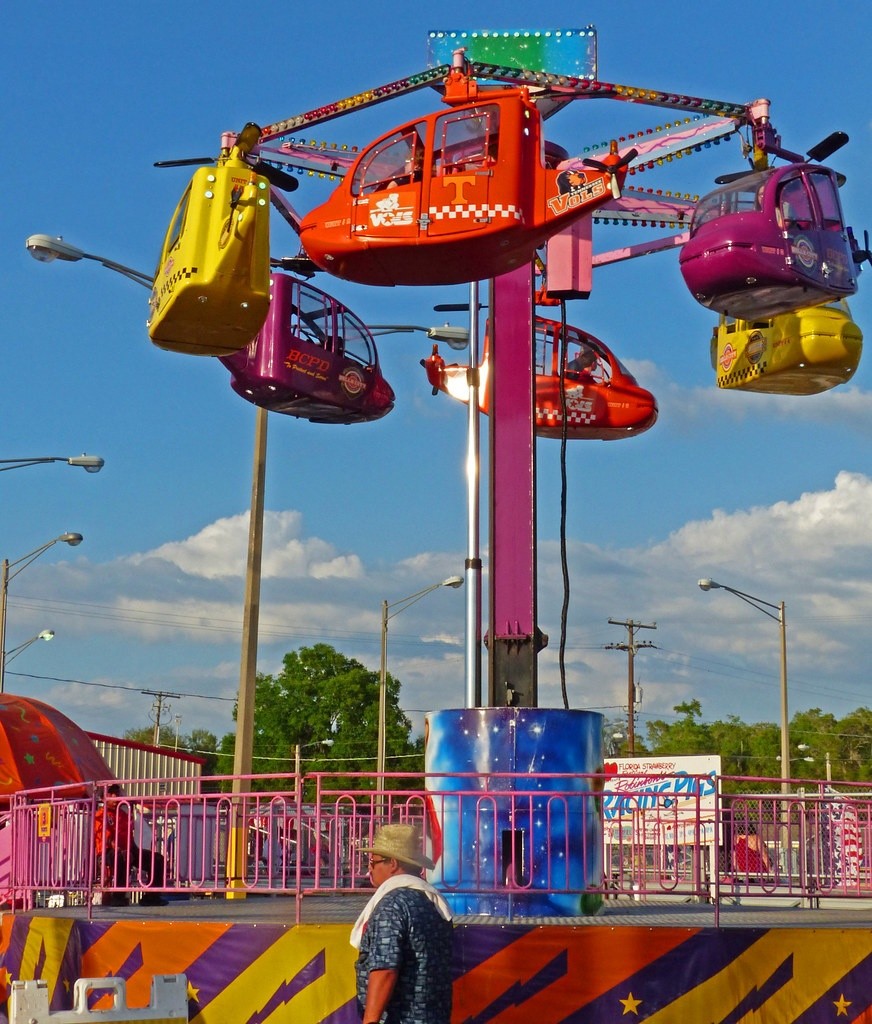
xmin=1 ymin=527 xmax=86 ymax=698
xmin=372 ymin=573 xmax=464 ymax=829
xmin=697 ymin=576 xmax=794 ymax=879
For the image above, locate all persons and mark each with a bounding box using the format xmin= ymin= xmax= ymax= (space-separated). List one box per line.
xmin=386 ymin=150 xmax=423 ymax=189
xmin=93 ymin=785 xmax=169 ymax=907
xmin=567 ymin=346 xmax=598 ymax=383
xmin=731 ymin=820 xmax=793 ymax=884
xmin=349 ymin=822 xmax=454 ymax=1024
xmin=755 ymin=184 xmax=782 ymax=229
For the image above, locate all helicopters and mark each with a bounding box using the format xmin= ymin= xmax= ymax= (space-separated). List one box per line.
xmin=709 ymin=296 xmax=865 ymax=398
xmin=147 ymin=121 xmax=300 ymax=359
xmin=219 ymin=251 xmax=397 ymax=426
xmin=301 ymin=89 xmax=639 ymax=286
xmin=417 ymin=302 xmax=658 ymax=441
xmin=680 ymin=130 xmax=872 ymax=324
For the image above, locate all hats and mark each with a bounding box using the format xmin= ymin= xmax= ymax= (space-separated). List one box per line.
xmin=355 ymin=824 xmax=435 ymax=870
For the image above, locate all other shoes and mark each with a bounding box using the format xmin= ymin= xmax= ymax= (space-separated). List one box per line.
xmin=111 ymin=892 xmax=129 ymax=906
xmin=138 ymin=895 xmax=169 ymax=906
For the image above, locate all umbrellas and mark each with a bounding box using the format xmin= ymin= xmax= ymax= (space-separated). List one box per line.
xmin=0 ymin=694 xmax=128 ymax=803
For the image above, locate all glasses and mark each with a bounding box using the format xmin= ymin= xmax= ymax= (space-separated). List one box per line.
xmin=370 ymin=858 xmax=390 ymax=869
xmin=758 ymin=192 xmax=764 ymax=198
